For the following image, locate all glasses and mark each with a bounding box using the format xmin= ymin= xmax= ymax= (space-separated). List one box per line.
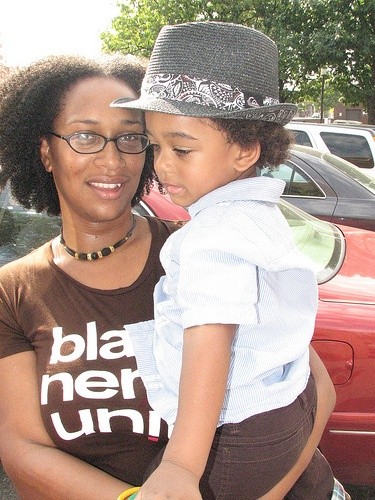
xmin=48 ymin=130 xmax=150 ymax=154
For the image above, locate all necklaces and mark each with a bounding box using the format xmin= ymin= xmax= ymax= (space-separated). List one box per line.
xmin=59 ymin=214 xmax=136 ymax=262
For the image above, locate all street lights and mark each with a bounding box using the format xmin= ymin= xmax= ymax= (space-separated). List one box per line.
xmin=319 ymin=66 xmax=328 ymax=124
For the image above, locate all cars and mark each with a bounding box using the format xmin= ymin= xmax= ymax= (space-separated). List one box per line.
xmin=134 ymin=177 xmax=375 ymax=492
xmin=255 ymin=120 xmax=375 ymax=233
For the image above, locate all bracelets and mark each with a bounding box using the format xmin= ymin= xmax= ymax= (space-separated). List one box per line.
xmin=117 ymin=487 xmax=142 ymax=500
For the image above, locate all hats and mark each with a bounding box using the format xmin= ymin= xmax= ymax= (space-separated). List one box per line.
xmin=110 ymin=22 xmax=297 ymax=126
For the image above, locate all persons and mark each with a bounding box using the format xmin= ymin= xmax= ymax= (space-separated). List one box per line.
xmin=0 ymin=59 xmax=336 ymax=500
xmin=109 ymin=23 xmax=351 ymax=500
